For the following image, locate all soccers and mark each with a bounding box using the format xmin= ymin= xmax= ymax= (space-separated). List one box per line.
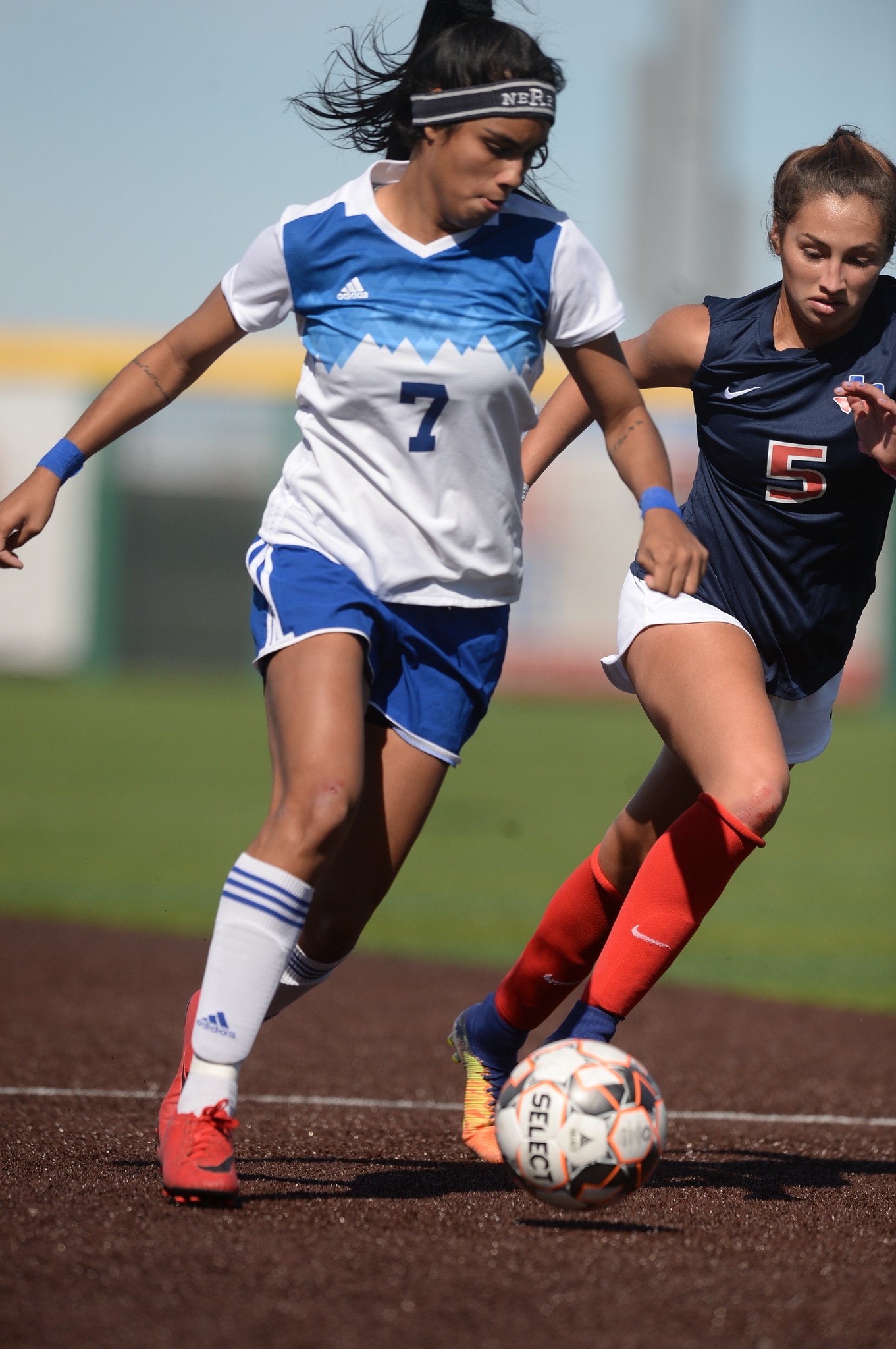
xmin=496 ymin=1038 xmax=665 ymax=1212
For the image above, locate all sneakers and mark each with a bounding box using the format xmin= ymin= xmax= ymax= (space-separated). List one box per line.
xmin=162 ymin=1106 xmax=241 ymax=1205
xmin=156 ymin=988 xmax=202 ymax=1165
xmin=447 ymin=1001 xmax=520 ymax=1165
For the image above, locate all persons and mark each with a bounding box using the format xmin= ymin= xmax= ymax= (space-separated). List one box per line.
xmin=1 ymin=0 xmax=707 ymax=1207
xmin=450 ymin=123 xmax=895 ymax=1174
